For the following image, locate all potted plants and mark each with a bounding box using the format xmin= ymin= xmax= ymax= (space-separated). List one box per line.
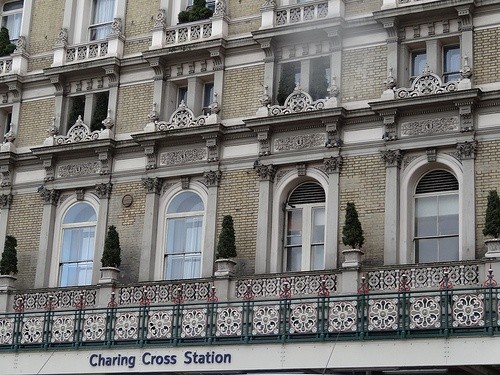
xmin=483 ymin=188 xmax=500 ymax=258
xmin=214 ymin=214 xmax=238 ymax=277
xmin=0 ymin=235 xmax=19 ymax=289
xmin=97 ymin=224 xmax=122 ymax=284
xmin=340 ymin=201 xmax=365 ymax=268
